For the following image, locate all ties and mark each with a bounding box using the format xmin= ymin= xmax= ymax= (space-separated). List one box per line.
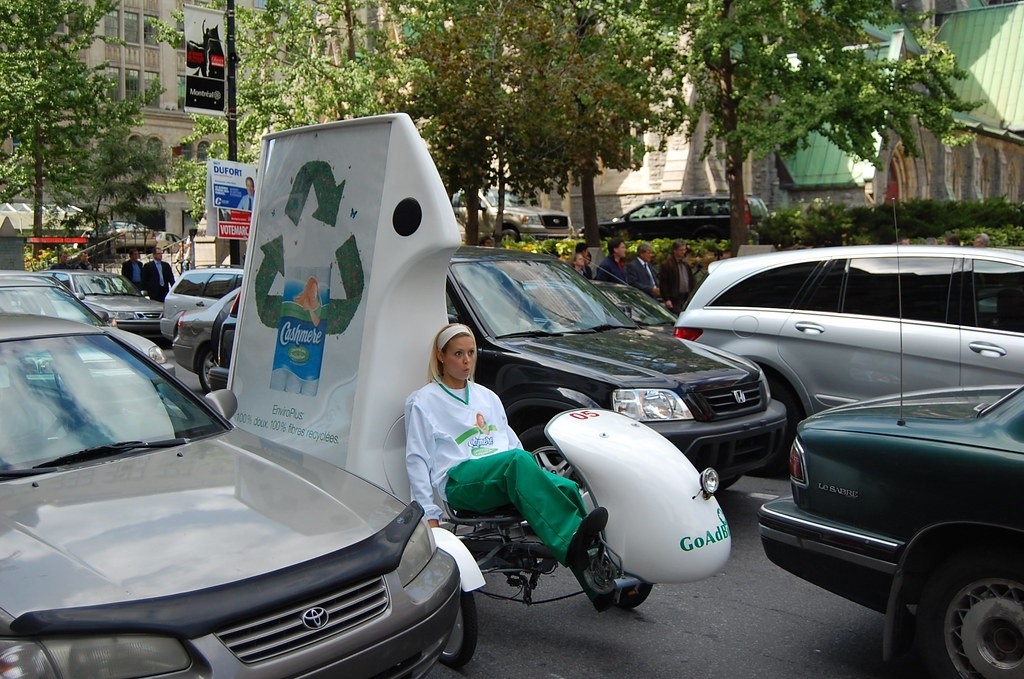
xmin=157 ymin=263 xmax=163 ymax=284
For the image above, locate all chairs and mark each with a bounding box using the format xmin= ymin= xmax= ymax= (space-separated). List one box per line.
xmin=443 ymin=502 xmax=526 ymax=527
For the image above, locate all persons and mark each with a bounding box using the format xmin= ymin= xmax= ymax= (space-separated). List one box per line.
xmin=625 ymin=244 xmax=661 ymax=297
xmin=72 ymin=252 xmax=93 ymax=270
xmin=238 ymin=177 xmax=254 ymax=211
xmin=594 ymin=238 xmax=628 ymax=284
xmin=401 ymin=321 xmax=608 ymax=570
xmin=658 ymin=240 xmax=696 ymax=315
xmin=895 ymin=232 xmax=990 ymax=250
xmin=477 ymin=237 xmax=495 ymax=246
xmin=50 ymin=252 xmax=74 ymax=271
xmin=141 ymin=248 xmax=176 ymax=303
xmin=570 ymin=241 xmax=591 ymax=280
xmin=121 ymin=248 xmax=144 ymax=293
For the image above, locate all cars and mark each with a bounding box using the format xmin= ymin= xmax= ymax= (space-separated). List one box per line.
xmin=0 ymin=269 xmax=175 ymax=424
xmin=39 ymin=269 xmax=172 ymax=349
xmin=674 ymin=245 xmax=1024 ymax=479
xmin=759 ymin=388 xmax=1024 ymax=679
xmin=156 ymin=232 xmax=182 ymax=253
xmin=0 ymin=311 xmax=461 ymax=678
xmin=172 ymin=285 xmax=240 ymax=392
xmin=85 ymin=220 xmax=157 ymax=254
xmin=518 ymin=278 xmax=678 ymax=339
xmin=448 ymin=187 xmax=575 ymax=243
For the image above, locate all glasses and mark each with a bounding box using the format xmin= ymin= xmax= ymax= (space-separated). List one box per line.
xmin=157 ymin=253 xmax=163 ymax=254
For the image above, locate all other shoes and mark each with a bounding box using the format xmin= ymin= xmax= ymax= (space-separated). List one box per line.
xmin=567 ymin=507 xmax=608 ymax=573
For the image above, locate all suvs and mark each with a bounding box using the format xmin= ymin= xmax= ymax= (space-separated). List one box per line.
xmin=159 ymin=264 xmax=244 ymax=341
xmin=206 ymin=245 xmax=789 ymax=492
xmin=576 ymin=194 xmax=769 ymax=243
